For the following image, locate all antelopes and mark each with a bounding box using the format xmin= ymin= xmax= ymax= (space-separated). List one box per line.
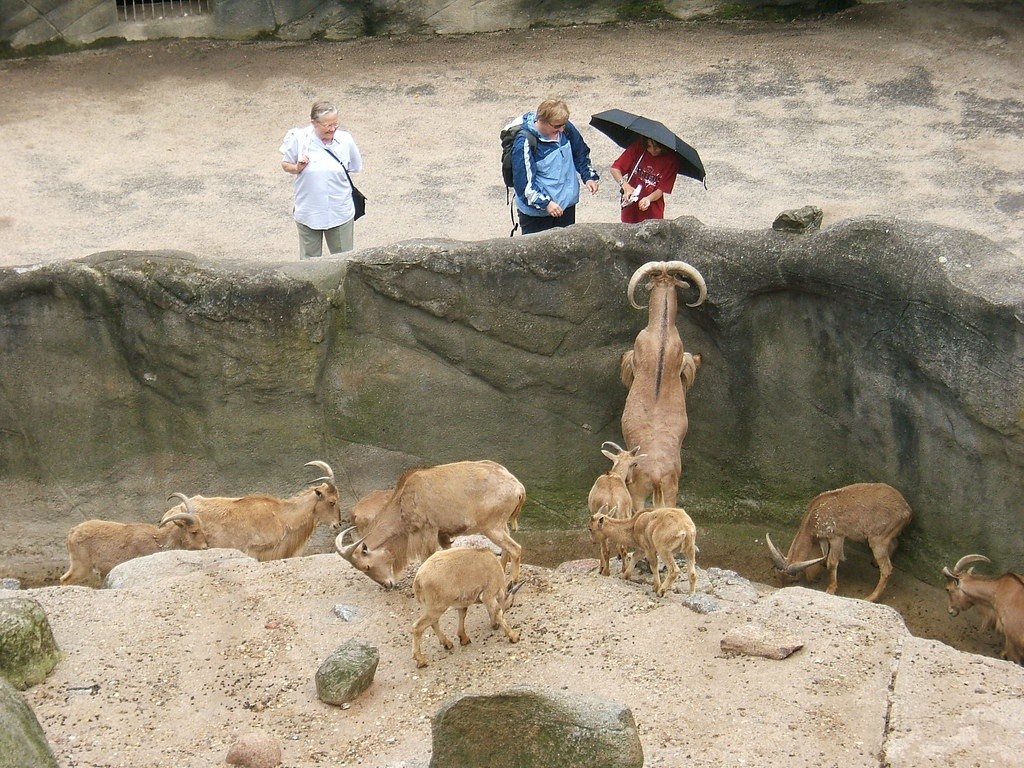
xmin=59 ymin=260 xmax=1024 ymax=669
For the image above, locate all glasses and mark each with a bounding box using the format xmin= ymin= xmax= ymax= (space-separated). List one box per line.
xmin=549 ymin=123 xmax=565 ymax=128
xmin=318 ymin=121 xmax=340 ymax=129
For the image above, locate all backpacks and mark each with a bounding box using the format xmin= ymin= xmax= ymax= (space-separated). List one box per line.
xmin=500 ymin=116 xmax=537 ymax=188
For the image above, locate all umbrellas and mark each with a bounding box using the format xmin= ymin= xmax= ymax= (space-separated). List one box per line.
xmin=589 ymin=108 xmax=708 ymax=194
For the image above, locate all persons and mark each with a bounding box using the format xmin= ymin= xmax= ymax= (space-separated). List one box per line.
xmin=509 ymin=99 xmax=599 ymax=235
xmin=610 ymin=137 xmax=678 ymax=224
xmin=281 ymin=100 xmax=362 ymax=260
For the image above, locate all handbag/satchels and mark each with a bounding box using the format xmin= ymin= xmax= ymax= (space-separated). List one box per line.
xmin=351 ymin=188 xmax=367 ymax=220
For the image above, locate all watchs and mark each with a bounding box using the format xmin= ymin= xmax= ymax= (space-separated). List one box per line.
xmin=618 ymin=179 xmax=625 ymax=186
